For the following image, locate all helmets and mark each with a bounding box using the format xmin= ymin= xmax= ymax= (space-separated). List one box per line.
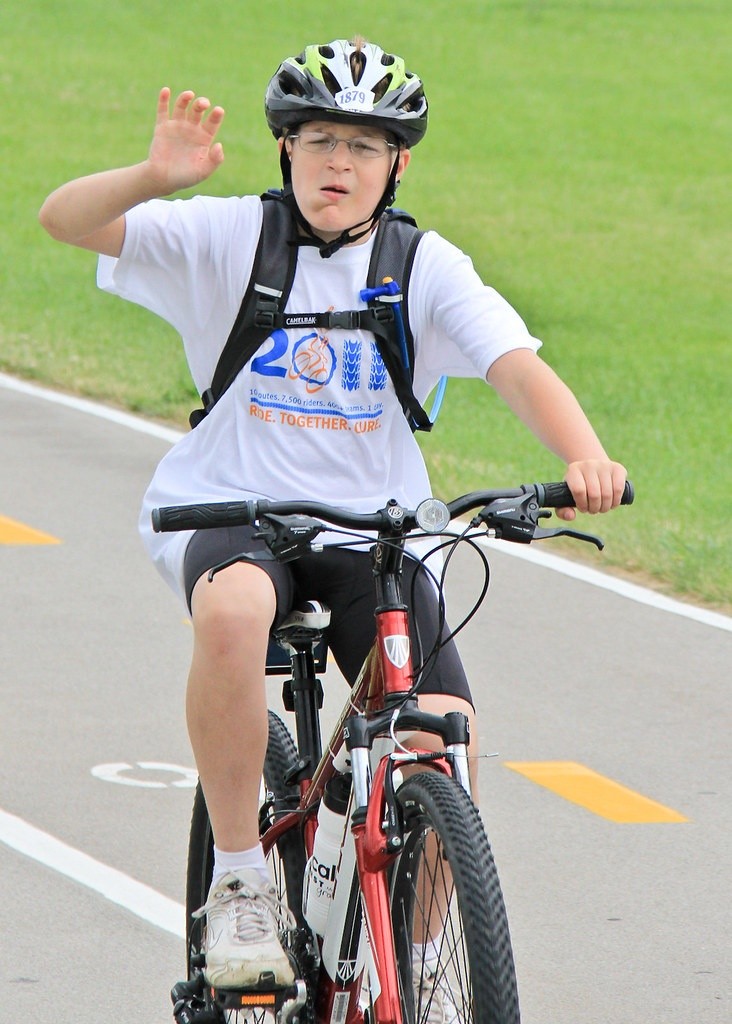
xmin=264 ymin=39 xmax=428 ymax=151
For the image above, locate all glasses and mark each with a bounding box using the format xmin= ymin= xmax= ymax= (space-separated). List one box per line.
xmin=284 ymin=130 xmax=399 ymax=159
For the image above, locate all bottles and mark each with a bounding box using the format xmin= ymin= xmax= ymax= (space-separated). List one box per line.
xmin=303 ymin=759 xmax=352 ymax=936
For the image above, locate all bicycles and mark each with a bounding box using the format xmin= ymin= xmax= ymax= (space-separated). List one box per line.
xmin=146 ymin=479 xmax=638 ymax=1023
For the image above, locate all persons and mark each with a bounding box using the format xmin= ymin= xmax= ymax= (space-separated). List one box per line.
xmin=38 ymin=27 xmax=629 ymax=1023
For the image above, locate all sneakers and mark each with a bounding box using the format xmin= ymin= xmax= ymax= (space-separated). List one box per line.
xmin=409 ymin=953 xmax=462 ymax=1024
xmin=200 ymin=867 xmax=299 ymax=989
